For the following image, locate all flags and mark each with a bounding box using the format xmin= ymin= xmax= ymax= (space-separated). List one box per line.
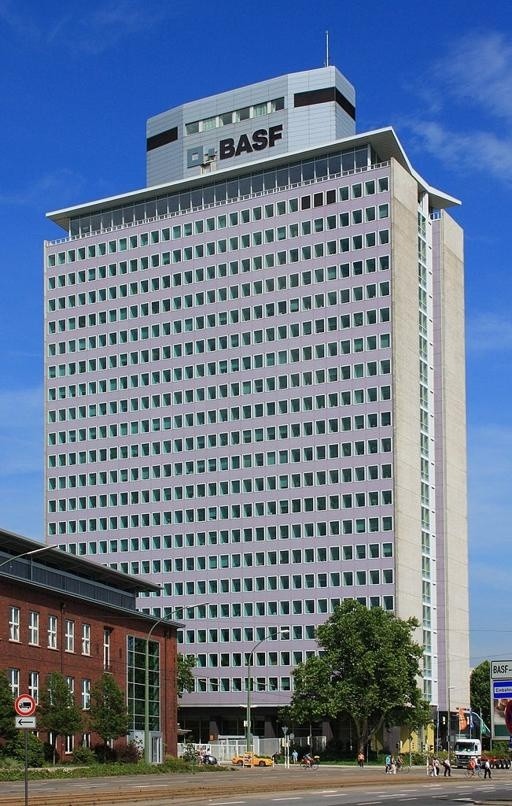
xmin=459 ymin=708 xmax=467 ymax=731
xmin=469 ymin=710 xmax=475 ymax=731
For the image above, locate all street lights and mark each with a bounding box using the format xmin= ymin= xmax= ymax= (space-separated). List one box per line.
xmin=246 ymin=629 xmax=289 ymax=750
xmin=0 ymin=543 xmax=58 ymax=568
xmin=144 ymin=601 xmax=209 ymax=764
xmin=447 ymin=686 xmax=456 ymax=760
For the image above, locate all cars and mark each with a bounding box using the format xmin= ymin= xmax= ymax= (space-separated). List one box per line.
xmin=180 ymin=752 xmax=273 ymax=767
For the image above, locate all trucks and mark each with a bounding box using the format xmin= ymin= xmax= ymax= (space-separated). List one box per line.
xmin=454 ymin=739 xmax=511 ymax=769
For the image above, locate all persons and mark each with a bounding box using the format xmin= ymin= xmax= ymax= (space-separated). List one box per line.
xmin=397 ymin=754 xmax=405 ymax=774
xmin=430 ymin=757 xmax=440 ymax=776
xmin=385 ymin=755 xmax=391 ymax=774
xmin=430 ymin=758 xmax=438 ymax=777
xmin=389 ymin=755 xmax=396 ymax=775
xmin=483 ymin=758 xmax=492 ymax=778
xmin=442 ymin=756 xmax=452 ymax=777
xmin=304 ymin=752 xmax=313 ymax=767
xmin=357 ymin=752 xmax=364 ymax=768
xmin=272 ymin=752 xmax=279 ymax=764
xmin=467 ymin=756 xmax=476 ymax=777
xmin=292 ymin=749 xmax=298 ymax=765
xmin=202 ymin=748 xmax=211 ymax=763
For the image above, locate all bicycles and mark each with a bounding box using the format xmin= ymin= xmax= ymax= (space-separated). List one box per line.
xmin=464 ymin=765 xmax=485 ymax=778
xmin=300 ymin=759 xmax=319 ymax=770
xmin=395 ymin=763 xmax=410 ymax=773
